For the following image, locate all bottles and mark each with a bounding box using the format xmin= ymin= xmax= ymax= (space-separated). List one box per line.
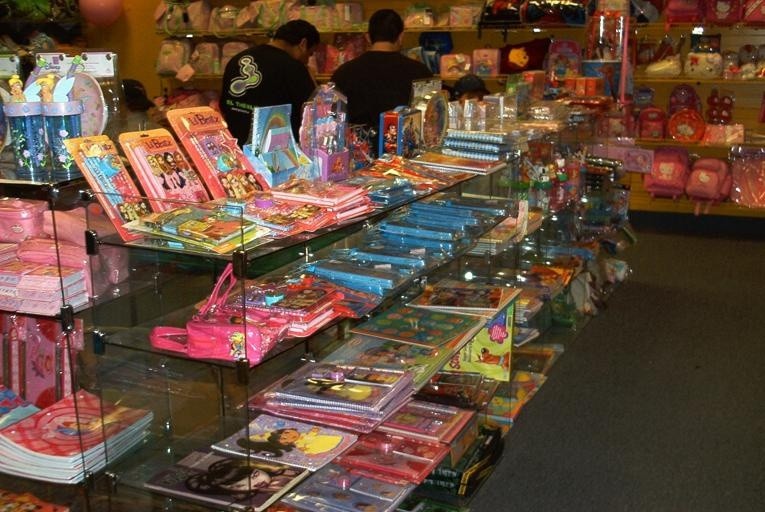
xmin=220 ymin=4 xmax=237 ymax=31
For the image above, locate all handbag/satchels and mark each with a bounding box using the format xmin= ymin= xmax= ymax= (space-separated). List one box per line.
xmin=148 ymin=262 xmax=291 ymax=369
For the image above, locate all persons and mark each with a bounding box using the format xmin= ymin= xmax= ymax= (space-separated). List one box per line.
xmin=118 ymin=78 xmax=174 ymax=136
xmin=450 ymin=74 xmax=491 ymax=104
xmin=218 ymin=18 xmax=320 ymax=150
xmin=331 ymin=8 xmax=433 ymax=158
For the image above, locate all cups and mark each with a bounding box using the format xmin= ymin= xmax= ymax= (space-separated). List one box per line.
xmin=3 ymin=99 xmax=46 ymax=178
xmin=40 ymin=98 xmax=88 ymax=178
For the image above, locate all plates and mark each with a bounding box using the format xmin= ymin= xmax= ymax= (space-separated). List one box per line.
xmin=72 ymin=72 xmax=109 ymax=137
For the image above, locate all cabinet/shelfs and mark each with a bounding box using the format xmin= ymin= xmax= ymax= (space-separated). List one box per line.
xmin=150 ymin=1 xmax=764 ymax=214
xmin=80 ymin=143 xmax=516 ymax=512
xmin=1 ymin=147 xmax=174 ymax=512
xmin=427 ymin=86 xmax=638 ymax=428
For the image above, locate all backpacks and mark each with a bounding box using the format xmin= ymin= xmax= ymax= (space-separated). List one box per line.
xmin=542 ymin=39 xmax=583 ymax=80
xmin=220 ymin=41 xmax=251 ymax=77
xmin=635 ymin=105 xmax=669 ymax=139
xmin=450 ymin=0 xmax=482 ymax=31
xmin=630 ymin=0 xmax=765 ymax=26
xmin=156 ymin=38 xmax=192 ymax=77
xmin=153 ymin=1 xmax=364 ymax=37
xmin=642 ymin=146 xmax=691 ymax=201
xmin=685 ymin=156 xmax=732 ymax=217
xmin=404 ymin=2 xmax=435 ymax=30
xmin=191 ymin=42 xmax=220 ymax=74
